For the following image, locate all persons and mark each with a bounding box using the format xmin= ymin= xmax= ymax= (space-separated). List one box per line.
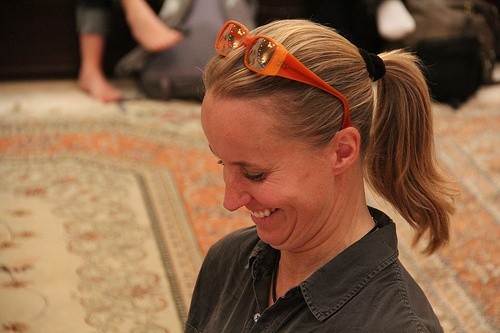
xmin=71 ymin=1 xmax=183 ymax=102
xmin=185 ymin=20 xmax=460 ymax=333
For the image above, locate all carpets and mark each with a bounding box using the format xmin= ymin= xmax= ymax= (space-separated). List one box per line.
xmin=2 ymin=63 xmax=500 ymax=333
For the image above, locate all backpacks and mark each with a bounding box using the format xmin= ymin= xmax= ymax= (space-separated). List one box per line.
xmin=115 ymin=0 xmax=258 ymax=99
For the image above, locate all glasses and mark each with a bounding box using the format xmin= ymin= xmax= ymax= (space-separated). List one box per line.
xmin=214 ymin=18 xmax=351 ymax=132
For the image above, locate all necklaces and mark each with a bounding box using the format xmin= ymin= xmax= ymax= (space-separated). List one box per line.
xmin=273 ymin=219 xmax=386 ymax=305
